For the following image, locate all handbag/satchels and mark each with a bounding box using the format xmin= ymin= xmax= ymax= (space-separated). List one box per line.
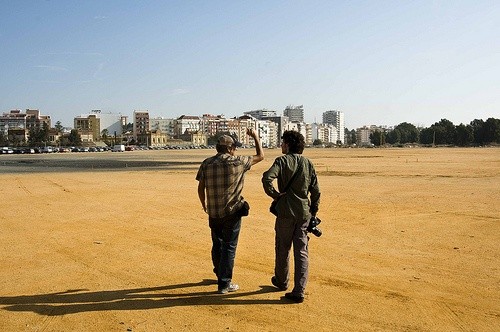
xmin=269 ymin=199 xmax=278 ymax=217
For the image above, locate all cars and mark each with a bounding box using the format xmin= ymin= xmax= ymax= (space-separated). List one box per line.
xmin=0 ymin=145 xmax=216 ymax=154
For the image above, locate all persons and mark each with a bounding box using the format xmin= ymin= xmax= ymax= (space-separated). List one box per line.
xmin=261 ymin=130 xmax=321 ymax=303
xmin=196 ymin=128 xmax=265 ymax=294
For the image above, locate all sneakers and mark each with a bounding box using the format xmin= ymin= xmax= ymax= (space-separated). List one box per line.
xmin=213 ymin=268 xmax=218 ymax=278
xmin=218 ymin=283 xmax=239 ymax=294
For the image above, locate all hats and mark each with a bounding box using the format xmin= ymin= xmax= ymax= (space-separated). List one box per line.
xmin=216 ymin=134 xmax=242 ymax=148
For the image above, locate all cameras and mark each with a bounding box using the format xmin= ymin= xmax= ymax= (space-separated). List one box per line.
xmin=307 ymin=217 xmax=322 ymax=237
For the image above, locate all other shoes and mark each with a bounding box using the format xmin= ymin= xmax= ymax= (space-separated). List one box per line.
xmin=285 ymin=292 xmax=304 ymax=303
xmin=271 ymin=276 xmax=288 ymax=291
